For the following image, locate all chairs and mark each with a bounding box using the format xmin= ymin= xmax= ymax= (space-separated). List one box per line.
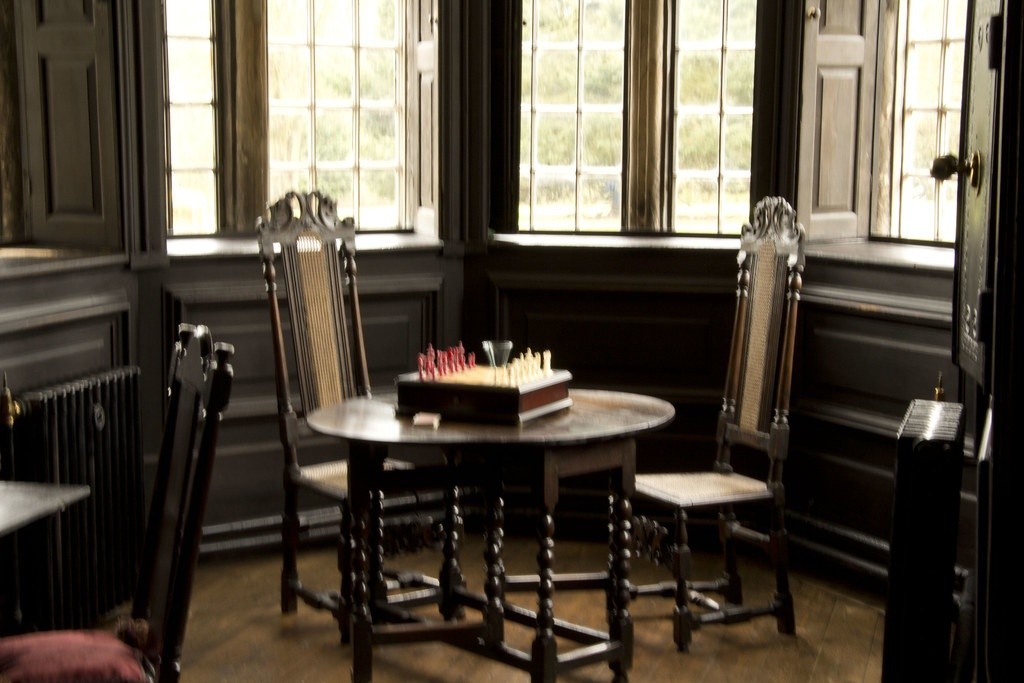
xmin=634 ymin=198 xmax=804 ymax=651
xmin=257 ymin=192 xmax=473 ymax=643
xmin=0 ymin=323 xmax=234 ymax=682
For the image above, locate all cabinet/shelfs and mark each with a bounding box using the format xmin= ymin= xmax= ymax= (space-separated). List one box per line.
xmin=883 ymin=1 xmax=1024 ymax=683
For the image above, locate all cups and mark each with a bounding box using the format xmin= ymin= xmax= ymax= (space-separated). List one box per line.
xmin=482 ymin=339 xmax=513 ymax=367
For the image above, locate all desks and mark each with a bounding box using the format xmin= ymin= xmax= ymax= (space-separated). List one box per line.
xmin=0 ymin=479 xmax=89 ymax=533
xmin=307 ymin=389 xmax=674 ymax=683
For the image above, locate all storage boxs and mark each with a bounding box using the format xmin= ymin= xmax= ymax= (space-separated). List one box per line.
xmin=396 ymin=365 xmax=574 ymax=423
